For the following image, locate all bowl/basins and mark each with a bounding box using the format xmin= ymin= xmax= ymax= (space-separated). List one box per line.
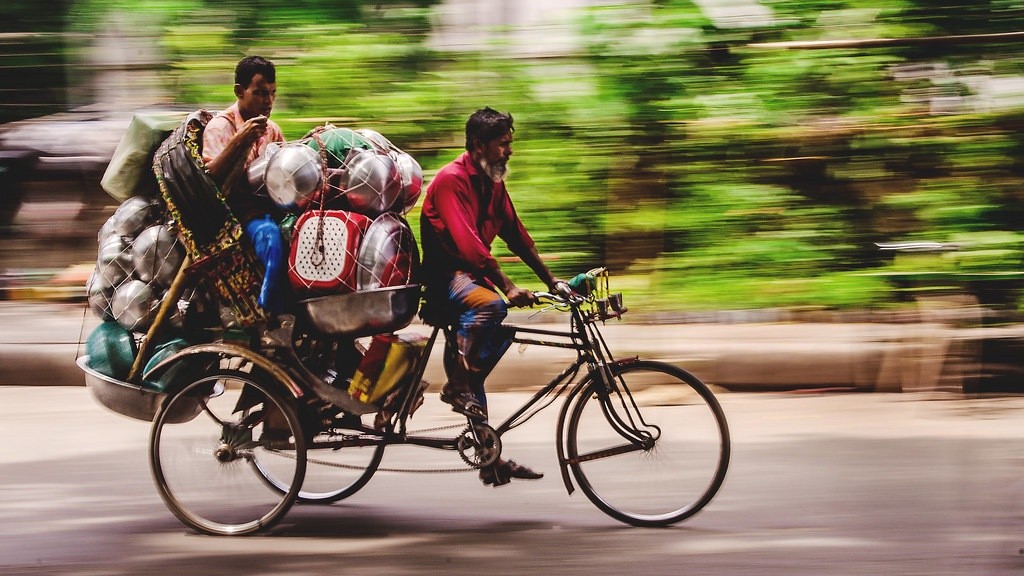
xmin=74 ymin=125 xmax=429 ymax=425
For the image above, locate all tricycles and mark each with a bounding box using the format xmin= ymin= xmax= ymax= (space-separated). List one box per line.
xmin=138 ymin=109 xmax=732 ymax=538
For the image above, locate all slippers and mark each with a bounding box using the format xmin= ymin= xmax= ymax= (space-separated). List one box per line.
xmin=440 ymin=391 xmax=487 ymax=419
xmin=483 ymin=460 xmax=544 ymax=484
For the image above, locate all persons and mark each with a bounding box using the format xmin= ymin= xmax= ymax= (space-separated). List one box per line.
xmin=419 ymin=105 xmax=568 ymax=486
xmin=202 ymin=53 xmax=290 ymax=315
